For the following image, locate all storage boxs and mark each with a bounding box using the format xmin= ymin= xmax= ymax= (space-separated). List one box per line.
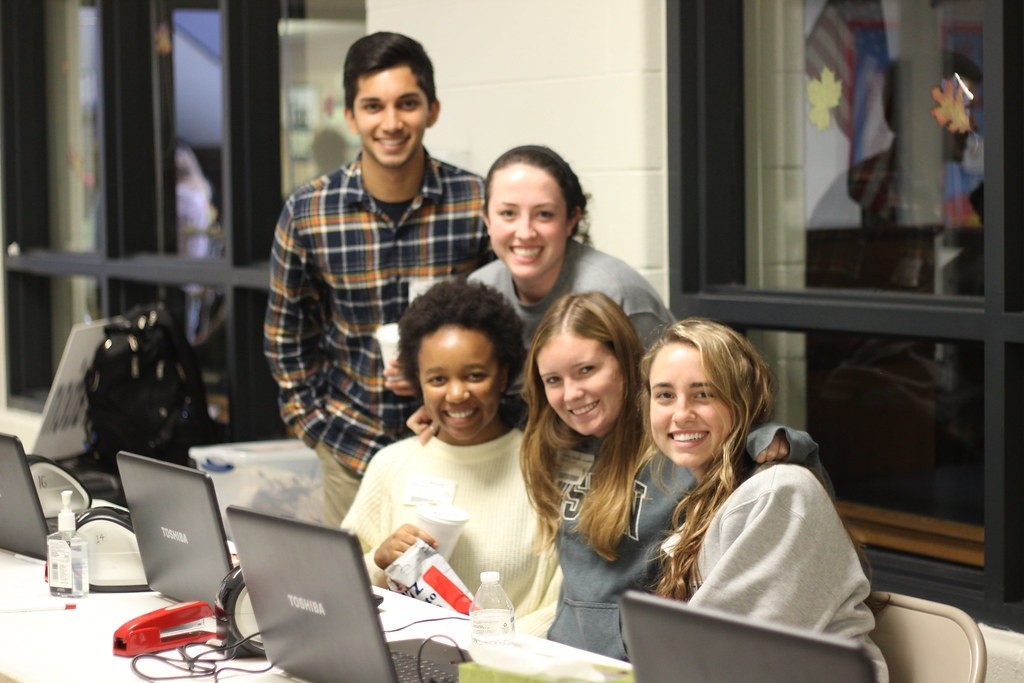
xmin=188 ymin=439 xmax=320 ymax=541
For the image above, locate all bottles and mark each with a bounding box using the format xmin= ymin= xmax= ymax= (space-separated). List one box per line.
xmin=468 ymin=571 xmax=516 ymax=667
xmin=46 ymin=490 xmax=89 ymax=597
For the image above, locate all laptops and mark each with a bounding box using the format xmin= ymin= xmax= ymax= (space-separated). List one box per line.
xmin=116 ymin=451 xmax=234 ymax=619
xmin=0 ymin=433 xmax=132 ymax=562
xmin=226 ymin=506 xmax=472 ymax=682
xmin=618 ymin=589 xmax=880 ymax=682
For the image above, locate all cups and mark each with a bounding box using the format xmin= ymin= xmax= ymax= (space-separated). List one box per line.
xmin=372 ymin=322 xmax=404 ymax=382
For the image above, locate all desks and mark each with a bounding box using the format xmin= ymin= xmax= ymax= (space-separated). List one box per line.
xmin=1 ymin=496 xmax=634 ymax=681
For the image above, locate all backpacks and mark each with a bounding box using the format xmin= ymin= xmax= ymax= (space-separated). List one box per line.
xmin=88 ymin=312 xmax=208 ymax=468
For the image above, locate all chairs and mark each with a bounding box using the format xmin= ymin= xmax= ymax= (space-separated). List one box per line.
xmin=870 ymin=591 xmax=988 ymax=683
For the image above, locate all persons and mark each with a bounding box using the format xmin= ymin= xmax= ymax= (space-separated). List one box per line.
xmin=806 ymin=46 xmax=977 ymax=297
xmin=330 ymin=277 xmax=566 ymax=620
xmin=264 ymin=31 xmax=496 ymax=527
xmin=639 ymin=317 xmax=896 ymax=681
xmin=520 ymin=287 xmax=821 ymax=668
xmin=463 ymin=145 xmax=679 ymax=378
xmin=169 ymin=139 xmax=216 ymax=360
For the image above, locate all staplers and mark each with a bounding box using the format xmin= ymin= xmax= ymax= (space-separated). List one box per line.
xmin=114 ymin=601 xmax=216 ymax=657
